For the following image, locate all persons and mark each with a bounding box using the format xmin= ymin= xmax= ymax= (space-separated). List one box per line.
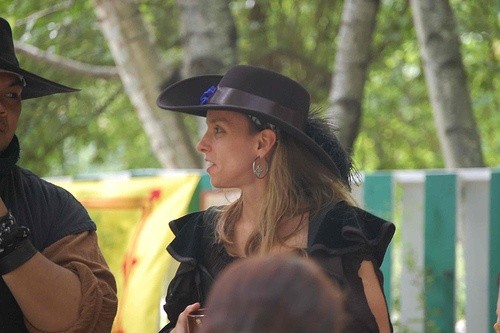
xmin=200 ymin=248 xmax=346 ymax=333
xmin=493 ymin=285 xmax=500 ymax=333
xmin=155 ymin=64 xmax=397 ymax=333
xmin=0 ymin=17 xmax=119 ymax=333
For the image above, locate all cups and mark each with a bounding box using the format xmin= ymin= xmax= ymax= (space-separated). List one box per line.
xmin=187 ymin=308 xmax=206 ymax=333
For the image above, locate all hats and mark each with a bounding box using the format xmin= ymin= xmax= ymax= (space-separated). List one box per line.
xmin=0 ymin=18 xmax=82 ymax=99
xmin=157 ymin=66 xmax=340 ymax=176
